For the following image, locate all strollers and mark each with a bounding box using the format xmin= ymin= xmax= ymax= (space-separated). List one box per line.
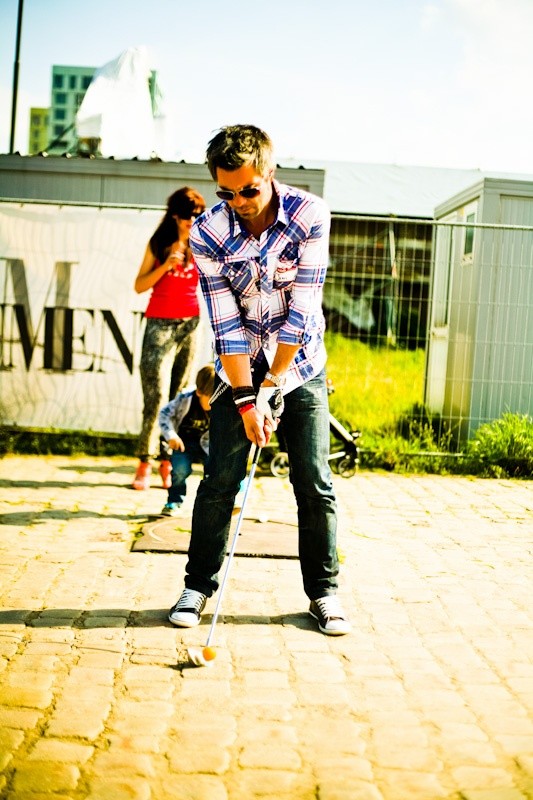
xmin=252 ymin=383 xmax=362 ymax=478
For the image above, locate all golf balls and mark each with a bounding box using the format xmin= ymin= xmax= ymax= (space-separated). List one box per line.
xmin=202 ymin=646 xmax=216 ymax=661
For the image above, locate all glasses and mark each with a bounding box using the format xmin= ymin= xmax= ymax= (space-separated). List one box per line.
xmin=176 ymin=211 xmax=203 ymax=221
xmin=214 ymin=176 xmax=268 ymax=201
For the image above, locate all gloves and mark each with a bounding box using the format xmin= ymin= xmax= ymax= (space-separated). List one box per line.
xmin=254 ymin=385 xmax=284 ymax=432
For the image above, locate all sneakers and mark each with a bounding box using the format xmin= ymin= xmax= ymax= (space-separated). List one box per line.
xmin=158 ymin=460 xmax=174 ymax=489
xmin=308 ymin=596 xmax=350 ymax=636
xmin=167 ymin=587 xmax=208 ymax=628
xmin=130 ymin=462 xmax=154 ymax=489
xmin=162 ymin=500 xmax=178 ymax=517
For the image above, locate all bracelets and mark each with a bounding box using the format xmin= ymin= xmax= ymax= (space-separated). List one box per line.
xmin=232 ymin=386 xmax=256 ymax=415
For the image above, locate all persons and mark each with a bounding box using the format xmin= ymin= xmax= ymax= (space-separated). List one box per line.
xmin=130 ymin=185 xmax=207 ymax=490
xmin=169 ymin=125 xmax=354 ymax=636
xmin=158 ymin=364 xmax=247 ymax=516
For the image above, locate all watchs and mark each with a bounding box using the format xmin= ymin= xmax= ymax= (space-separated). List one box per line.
xmin=264 ymin=372 xmax=287 ymax=387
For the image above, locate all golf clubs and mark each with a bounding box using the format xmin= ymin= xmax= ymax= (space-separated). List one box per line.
xmin=186 ymin=389 xmax=283 ymax=667
